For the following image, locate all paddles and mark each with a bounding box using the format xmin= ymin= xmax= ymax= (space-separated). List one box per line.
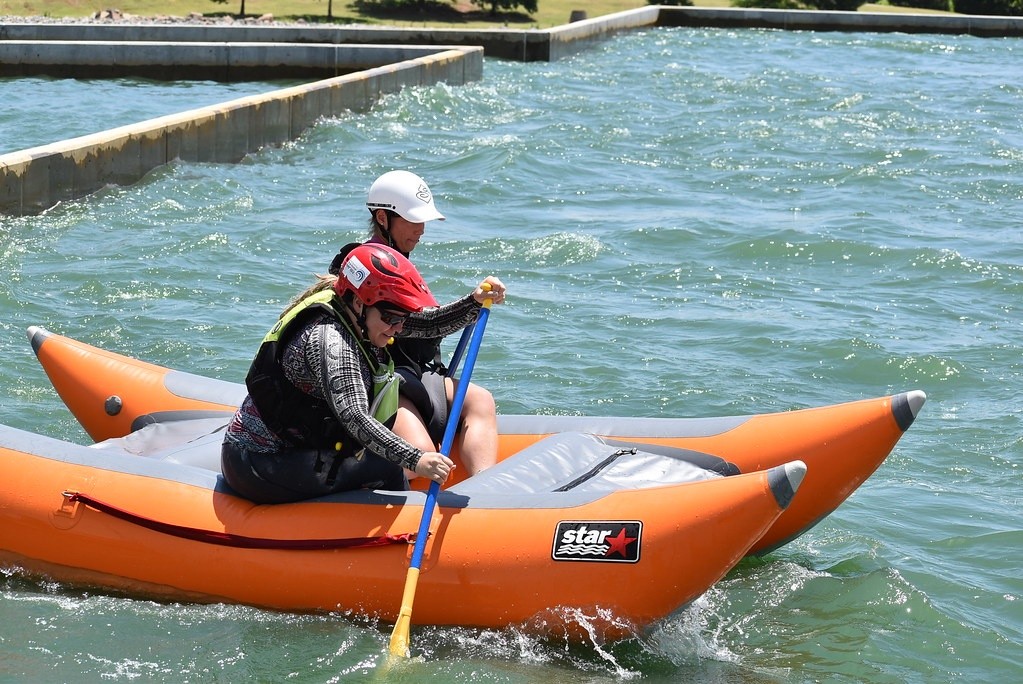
xmin=387 ymin=283 xmax=492 ymax=658
xmin=446 ymin=322 xmax=476 ymax=378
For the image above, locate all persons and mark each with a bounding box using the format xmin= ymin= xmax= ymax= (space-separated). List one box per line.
xmin=220 ymin=244 xmax=506 ymax=506
xmin=328 ymin=170 xmax=497 ymax=481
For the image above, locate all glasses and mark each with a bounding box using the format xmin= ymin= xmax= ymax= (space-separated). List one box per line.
xmin=372 ymin=306 xmax=409 ymax=325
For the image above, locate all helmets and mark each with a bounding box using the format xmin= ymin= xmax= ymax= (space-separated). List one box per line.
xmin=333 ymin=243 xmax=440 ymax=314
xmin=366 ymin=170 xmax=445 ymax=223
